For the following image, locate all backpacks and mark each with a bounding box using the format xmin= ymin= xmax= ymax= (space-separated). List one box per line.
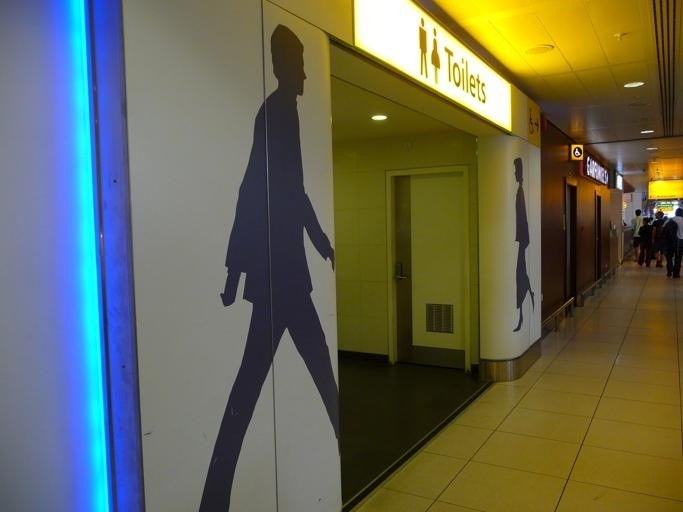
xmin=659 ymin=218 xmax=678 ymax=244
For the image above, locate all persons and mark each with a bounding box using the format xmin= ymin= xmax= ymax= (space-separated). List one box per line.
xmin=630 ymin=208 xmax=683 ymax=279
xmin=512 ymin=157 xmax=535 ymax=332
xmin=196 ymin=24 xmax=342 ymax=512
